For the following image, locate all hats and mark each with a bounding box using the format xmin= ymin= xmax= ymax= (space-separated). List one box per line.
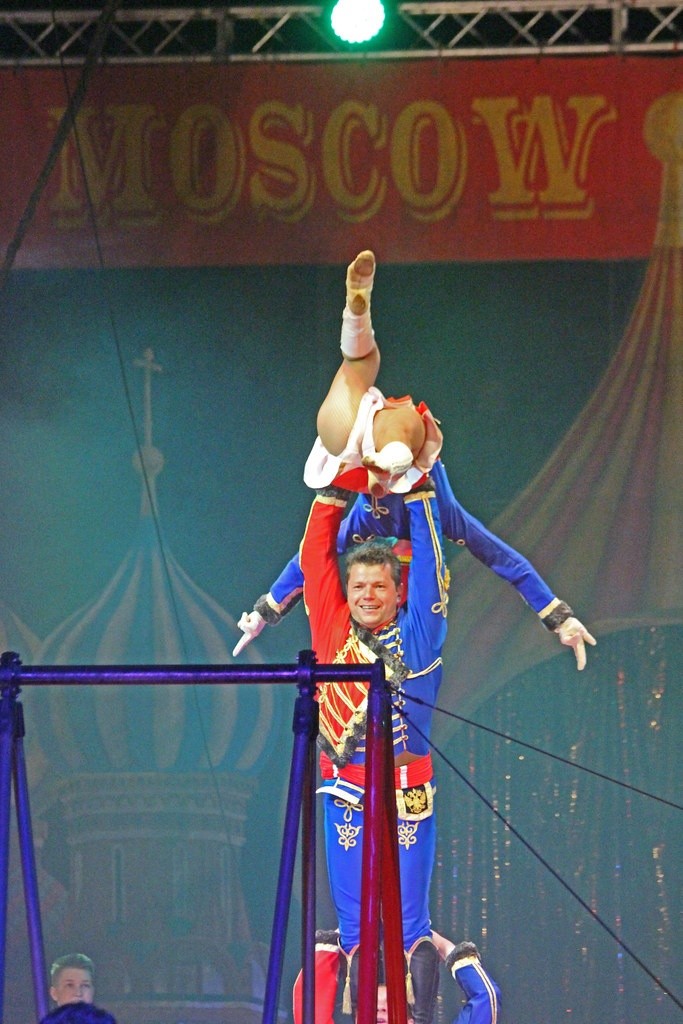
xmin=391 ymin=540 xmax=414 ymax=607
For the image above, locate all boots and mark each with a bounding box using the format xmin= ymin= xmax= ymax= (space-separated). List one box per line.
xmin=361 ymin=440 xmax=416 ymax=499
xmin=332 ymin=937 xmax=361 ymax=1024
xmin=404 ymin=936 xmax=439 ymax=1024
xmin=341 ymin=250 xmax=377 ymax=359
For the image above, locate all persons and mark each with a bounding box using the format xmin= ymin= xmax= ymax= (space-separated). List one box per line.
xmin=293 ymin=927 xmax=502 ymax=1024
xmin=233 ymin=249 xmax=600 ymax=672
xmin=298 ymin=473 xmax=445 ymax=1024
xmin=37 ymin=954 xmax=119 ymax=1024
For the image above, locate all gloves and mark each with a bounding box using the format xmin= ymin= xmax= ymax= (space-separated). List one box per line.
xmin=233 ymin=610 xmax=266 ymax=657
xmin=555 ymin=617 xmax=596 ymax=671
